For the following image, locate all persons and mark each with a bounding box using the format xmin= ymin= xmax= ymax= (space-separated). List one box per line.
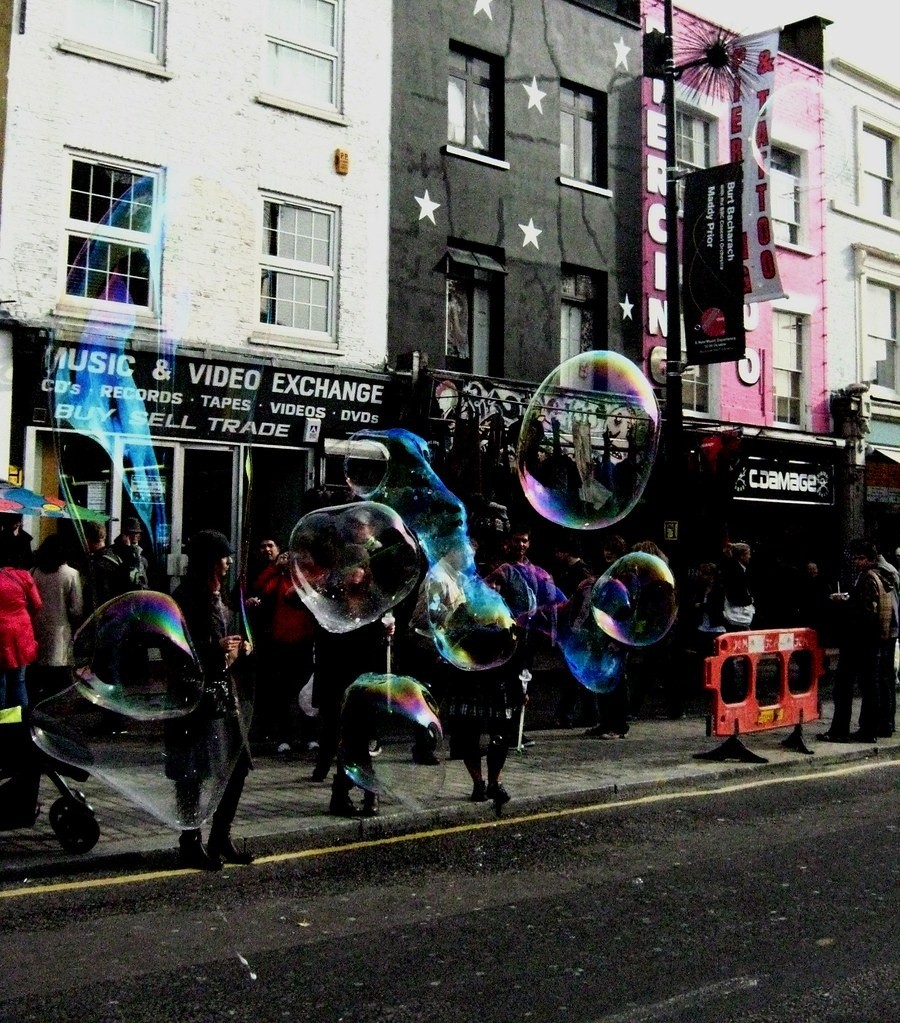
xmin=245 ymin=509 xmax=396 ymax=817
xmin=94 ymin=517 xmax=148 ymax=603
xmin=164 ymin=530 xmax=255 ymax=871
xmin=688 ymin=542 xmax=756 ymax=711
xmin=796 ymin=561 xmax=830 ymax=653
xmin=815 ymin=533 xmax=900 ymax=743
xmin=1 ymin=514 xmax=105 ymax=716
xmin=404 ymin=524 xmax=687 ymax=803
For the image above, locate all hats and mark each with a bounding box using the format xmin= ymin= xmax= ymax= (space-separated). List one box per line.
xmin=123 ymin=518 xmax=143 ymax=533
xmin=188 ymin=530 xmax=234 ymax=560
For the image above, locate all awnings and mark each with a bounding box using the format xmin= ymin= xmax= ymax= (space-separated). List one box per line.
xmin=2 ymin=488 xmax=112 ymax=522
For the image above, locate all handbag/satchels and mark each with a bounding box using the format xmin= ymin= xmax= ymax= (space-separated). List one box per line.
xmin=723 ymin=603 xmax=755 ymax=626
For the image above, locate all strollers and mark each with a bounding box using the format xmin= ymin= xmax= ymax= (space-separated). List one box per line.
xmin=1 ymin=705 xmax=99 ymax=853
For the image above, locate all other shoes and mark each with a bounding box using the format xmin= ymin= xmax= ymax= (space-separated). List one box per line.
xmin=487 ymin=782 xmax=511 ymax=801
xmin=267 ymin=735 xmax=291 ymax=752
xmin=470 ymin=781 xmax=488 ymax=801
xmin=362 ymin=792 xmax=380 ymax=815
xmin=584 ymin=722 xmax=629 ymax=741
xmin=294 ymin=736 xmax=321 ymax=751
xmin=329 ymin=795 xmax=356 ymax=816
xmin=815 ymin=717 xmax=896 ymax=743
xmin=412 ymin=745 xmax=439 ymax=764
xmin=508 ymin=732 xmax=536 ymax=750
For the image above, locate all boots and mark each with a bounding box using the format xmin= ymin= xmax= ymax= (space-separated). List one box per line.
xmin=182 ymin=828 xmax=223 ymax=870
xmin=208 ymin=819 xmax=252 ymax=864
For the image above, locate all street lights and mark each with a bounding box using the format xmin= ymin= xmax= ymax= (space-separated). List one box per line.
xmin=642 ymin=27 xmax=730 ymax=716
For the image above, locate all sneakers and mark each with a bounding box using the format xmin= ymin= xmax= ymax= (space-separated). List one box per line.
xmin=367 ymin=737 xmax=383 ymax=757
